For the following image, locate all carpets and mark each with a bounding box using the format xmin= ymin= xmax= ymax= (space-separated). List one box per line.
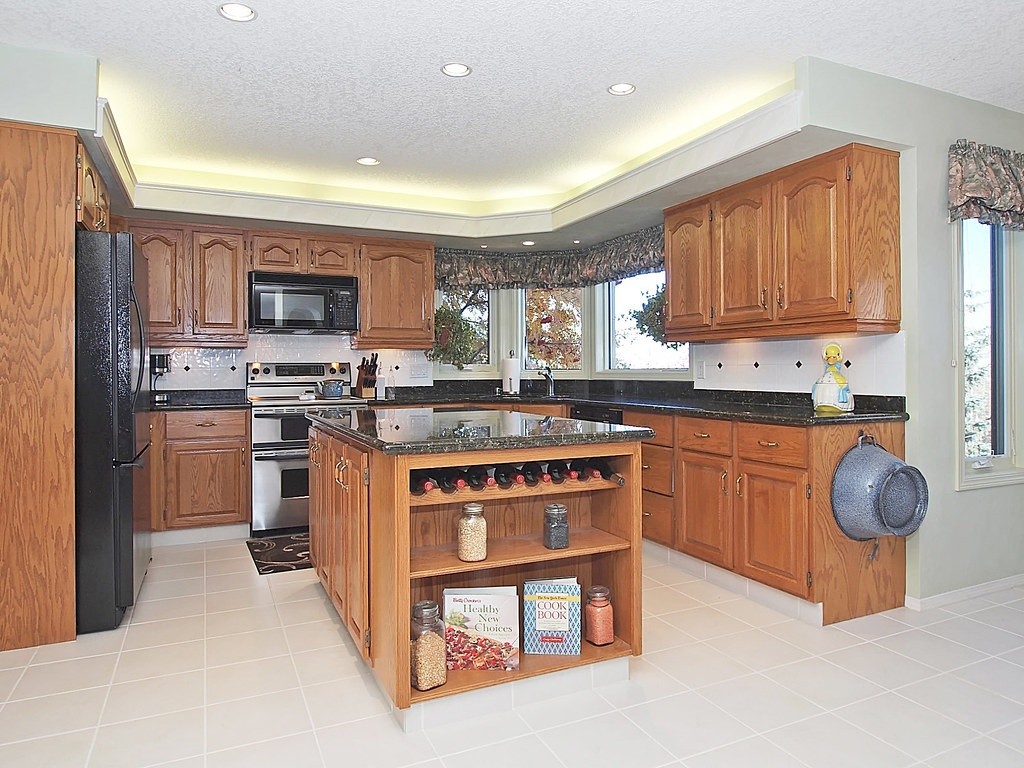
xmin=246 ymin=534 xmax=312 ymax=576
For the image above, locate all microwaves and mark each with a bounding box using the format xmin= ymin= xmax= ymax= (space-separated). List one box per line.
xmin=247 ymin=271 xmax=358 ymax=335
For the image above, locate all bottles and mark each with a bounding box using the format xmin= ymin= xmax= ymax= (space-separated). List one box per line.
xmin=376 ymin=362 xmax=385 ymax=400
xmin=386 ymin=365 xmax=396 ymax=400
xmin=409 ymin=458 xmax=624 ymax=496
xmin=543 ymin=503 xmax=570 ymax=550
xmin=410 ymin=599 xmax=447 ymax=692
xmin=456 ymin=503 xmax=488 ymax=563
xmin=584 ymin=584 xmax=615 ymax=647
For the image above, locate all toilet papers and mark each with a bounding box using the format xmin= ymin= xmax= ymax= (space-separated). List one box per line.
xmin=503 ymin=358 xmax=521 ymax=394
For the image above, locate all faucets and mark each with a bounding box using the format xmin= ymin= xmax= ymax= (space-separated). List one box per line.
xmin=537 ymin=366 xmax=557 ymax=399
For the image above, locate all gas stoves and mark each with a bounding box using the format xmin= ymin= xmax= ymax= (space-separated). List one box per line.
xmin=244 ymin=361 xmax=368 ymax=406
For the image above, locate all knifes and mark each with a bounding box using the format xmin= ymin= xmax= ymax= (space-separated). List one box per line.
xmin=360 ymin=353 xmax=378 ymax=388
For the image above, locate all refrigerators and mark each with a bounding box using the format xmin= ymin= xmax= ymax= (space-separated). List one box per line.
xmin=74 ymin=228 xmax=152 ymax=635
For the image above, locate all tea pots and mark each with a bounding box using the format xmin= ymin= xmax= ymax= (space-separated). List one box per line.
xmin=317 ymin=379 xmax=344 ymax=400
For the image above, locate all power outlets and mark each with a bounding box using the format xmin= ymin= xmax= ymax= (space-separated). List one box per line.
xmin=697 ymin=361 xmax=705 ymax=380
xmin=156 ymin=373 xmax=168 ymax=380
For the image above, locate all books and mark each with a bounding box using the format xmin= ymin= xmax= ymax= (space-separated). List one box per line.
xmin=522 ymin=576 xmax=582 ymax=656
xmin=442 ymin=585 xmax=519 ymax=671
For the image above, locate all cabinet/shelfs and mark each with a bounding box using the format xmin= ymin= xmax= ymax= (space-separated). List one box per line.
xmin=304 ymin=409 xmax=655 ymax=729
xmin=149 ymin=408 xmax=251 ymax=550
xmin=665 ymin=142 xmax=901 ymax=342
xmin=109 ymin=215 xmax=436 ymax=349
xmin=622 ymin=410 xmax=906 ymax=627
xmin=76 ymin=144 xmax=111 ymax=232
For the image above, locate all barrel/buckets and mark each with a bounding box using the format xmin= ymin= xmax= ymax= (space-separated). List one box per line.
xmin=830 ymin=433 xmax=929 ymax=542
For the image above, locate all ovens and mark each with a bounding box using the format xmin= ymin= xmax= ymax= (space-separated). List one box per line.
xmin=251 ymin=407 xmax=351 ymax=531
